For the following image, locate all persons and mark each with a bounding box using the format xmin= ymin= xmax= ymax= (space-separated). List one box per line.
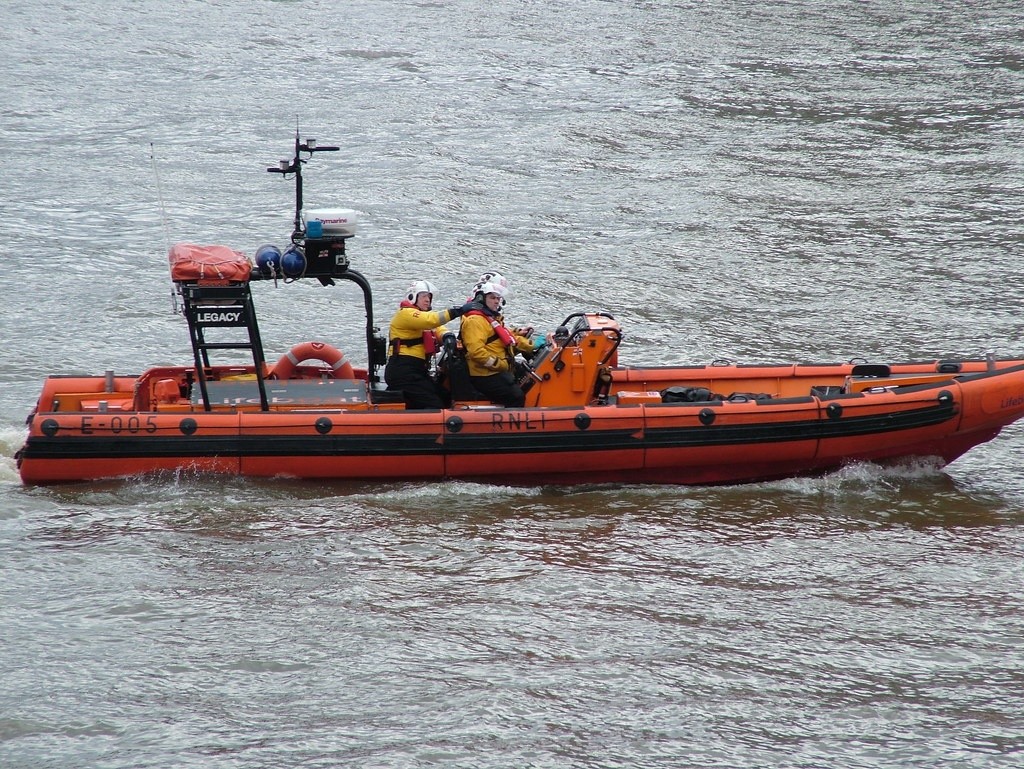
xmin=384 ymin=271 xmax=548 ymax=409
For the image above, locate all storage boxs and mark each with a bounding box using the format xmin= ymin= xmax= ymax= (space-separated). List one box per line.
xmin=616 ymin=392 xmax=663 ymax=403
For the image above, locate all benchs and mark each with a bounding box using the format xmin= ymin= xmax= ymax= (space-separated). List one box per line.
xmin=81 ymin=398 xmax=133 ymax=413
xmin=369 ymin=379 xmax=406 ymax=411
xmin=455 ymin=394 xmax=504 ymax=412
xmin=847 ymin=371 xmax=986 ymax=394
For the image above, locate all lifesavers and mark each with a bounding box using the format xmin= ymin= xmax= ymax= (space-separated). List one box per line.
xmin=270 ymin=344 xmax=356 ymax=380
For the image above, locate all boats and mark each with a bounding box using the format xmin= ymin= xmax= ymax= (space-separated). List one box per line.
xmin=11 ymin=113 xmax=1024 ymax=490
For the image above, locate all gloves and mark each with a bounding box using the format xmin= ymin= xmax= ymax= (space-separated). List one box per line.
xmin=443 ymin=335 xmax=457 ymax=354
xmin=461 ymin=301 xmax=483 ymax=314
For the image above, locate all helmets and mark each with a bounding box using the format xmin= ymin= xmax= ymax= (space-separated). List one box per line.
xmin=478 ymin=271 xmax=506 ymax=287
xmin=406 ymin=280 xmax=434 ymax=306
xmin=472 ymin=281 xmax=502 ymax=300
